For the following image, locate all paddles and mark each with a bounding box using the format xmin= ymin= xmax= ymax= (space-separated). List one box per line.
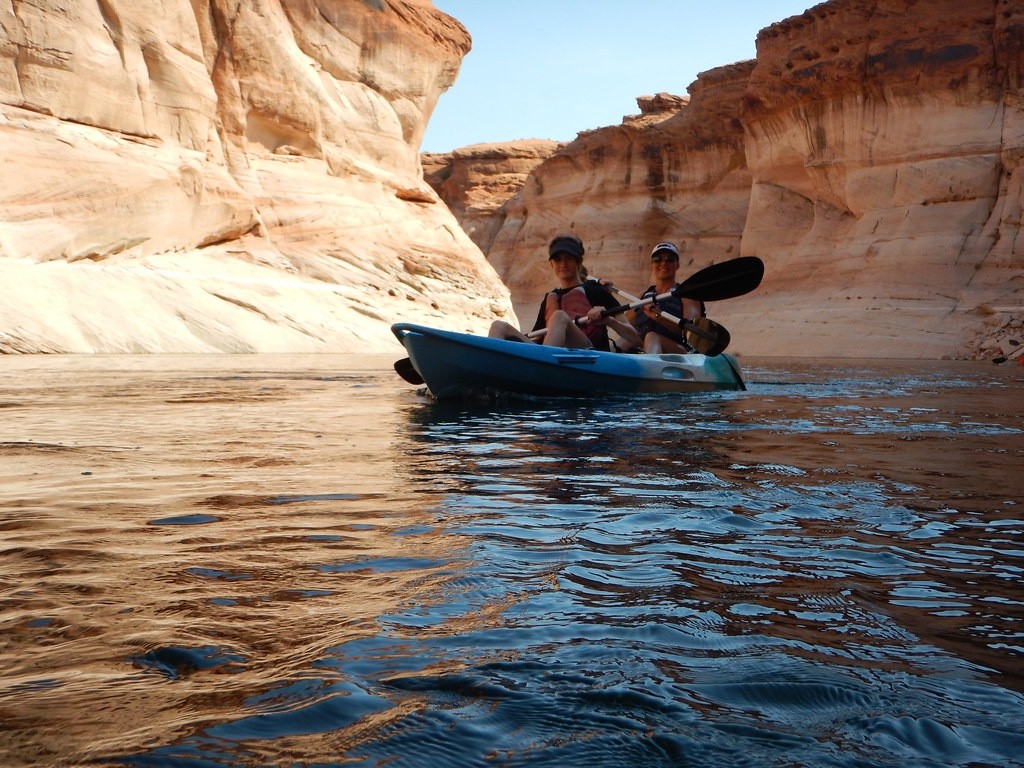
xmin=393 ymin=255 xmax=765 ymax=386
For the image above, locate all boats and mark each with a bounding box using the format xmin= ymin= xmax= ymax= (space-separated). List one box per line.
xmin=388 ymin=320 xmax=748 ymax=400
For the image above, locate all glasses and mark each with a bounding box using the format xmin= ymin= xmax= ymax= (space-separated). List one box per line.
xmin=651 ymin=256 xmax=674 ymax=263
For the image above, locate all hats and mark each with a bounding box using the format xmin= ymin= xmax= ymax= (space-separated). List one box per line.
xmin=548 ymin=234 xmax=584 ymax=260
xmin=651 ymin=241 xmax=681 ymax=260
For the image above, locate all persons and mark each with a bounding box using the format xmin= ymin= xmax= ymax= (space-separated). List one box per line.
xmin=598 ymin=241 xmax=702 ymax=354
xmin=489 ymin=234 xmax=642 ymax=347
